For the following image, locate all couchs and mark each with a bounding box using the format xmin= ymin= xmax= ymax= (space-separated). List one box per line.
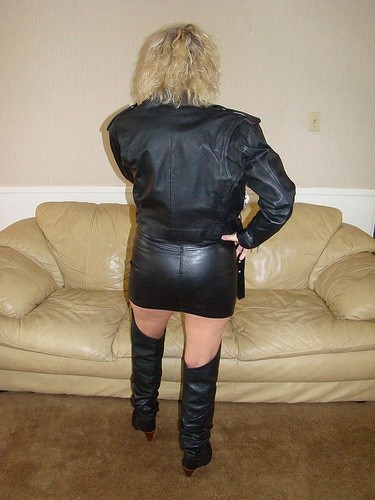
xmin=0 ymin=201 xmax=375 ymax=403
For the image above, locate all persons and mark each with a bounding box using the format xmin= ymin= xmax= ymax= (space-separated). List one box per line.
xmin=106 ymin=23 xmax=296 ymax=478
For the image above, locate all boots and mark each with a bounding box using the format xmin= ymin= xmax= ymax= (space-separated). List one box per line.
xmin=130 ymin=314 xmax=167 ymax=441
xmin=178 ymin=340 xmax=223 ymax=477
